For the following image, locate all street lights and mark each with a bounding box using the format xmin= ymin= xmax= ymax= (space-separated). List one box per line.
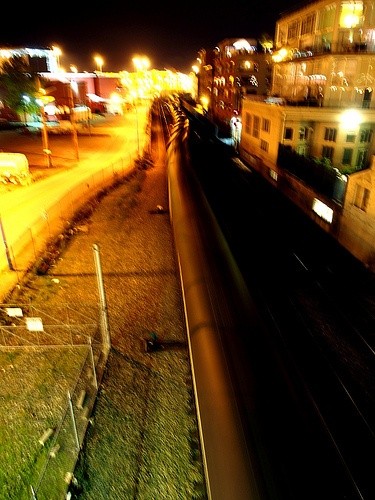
xmin=35 ymin=99 xmax=53 ymax=168
